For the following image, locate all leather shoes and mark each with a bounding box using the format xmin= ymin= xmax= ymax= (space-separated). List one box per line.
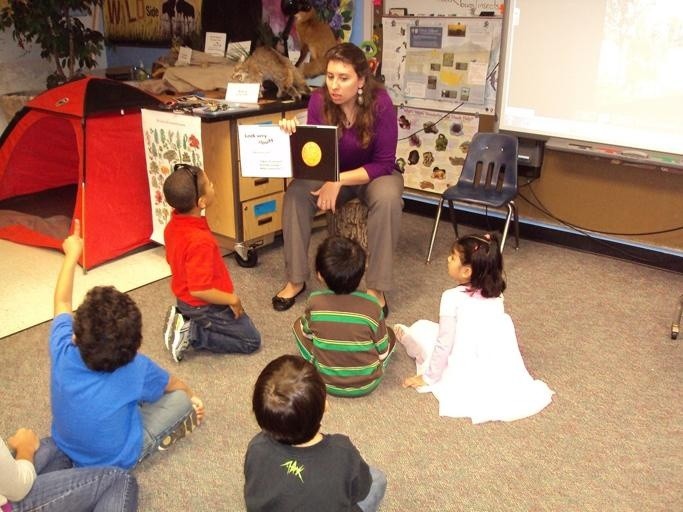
xmin=271 ymin=281 xmax=306 ymax=312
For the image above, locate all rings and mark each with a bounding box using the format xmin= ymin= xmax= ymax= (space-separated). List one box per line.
xmin=322 ymin=201 xmax=326 ymax=204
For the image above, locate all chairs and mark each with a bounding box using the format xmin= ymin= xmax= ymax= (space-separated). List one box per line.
xmin=426 ymin=133 xmax=520 ymax=263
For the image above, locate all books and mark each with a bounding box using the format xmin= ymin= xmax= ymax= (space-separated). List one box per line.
xmin=232 ymin=123 xmax=340 ymax=184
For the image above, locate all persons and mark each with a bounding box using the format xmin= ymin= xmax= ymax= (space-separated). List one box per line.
xmin=290 ymin=234 xmax=397 ymax=400
xmin=46 ymin=217 xmax=206 ymax=476
xmin=0 ymin=420 xmax=141 ymax=511
xmin=392 ymin=229 xmax=555 ymax=428
xmin=242 ymin=354 xmax=387 ymax=511
xmin=268 ymin=40 xmax=406 ymax=316
xmin=159 ymin=161 xmax=262 ymax=363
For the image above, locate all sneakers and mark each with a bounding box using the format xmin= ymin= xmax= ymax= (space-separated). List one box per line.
xmin=392 ymin=323 xmax=409 ymax=342
xmin=156 ymin=404 xmax=201 ymax=451
xmin=163 ymin=305 xmax=191 ymax=365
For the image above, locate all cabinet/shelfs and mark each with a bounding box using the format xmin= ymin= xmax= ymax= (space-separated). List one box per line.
xmin=140 ymin=92 xmax=314 ymax=266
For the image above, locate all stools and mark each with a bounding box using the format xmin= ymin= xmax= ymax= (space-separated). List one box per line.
xmin=325 ymin=199 xmax=372 ymax=267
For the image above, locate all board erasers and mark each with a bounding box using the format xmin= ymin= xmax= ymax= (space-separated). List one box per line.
xmin=622 ymin=150 xmax=647 ymax=159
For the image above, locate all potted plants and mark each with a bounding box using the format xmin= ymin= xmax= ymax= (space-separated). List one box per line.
xmin=0 ymin=0 xmax=105 ymax=91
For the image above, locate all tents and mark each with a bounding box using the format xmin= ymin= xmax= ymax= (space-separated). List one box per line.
xmin=0 ymin=75 xmax=172 ymax=278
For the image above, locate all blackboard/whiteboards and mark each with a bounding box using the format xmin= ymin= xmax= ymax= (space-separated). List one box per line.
xmin=494 ymin=0 xmax=683 ymax=173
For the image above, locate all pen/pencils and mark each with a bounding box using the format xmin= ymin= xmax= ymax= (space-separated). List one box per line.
xmin=650 ymin=154 xmax=679 ymax=162
xmin=569 ymin=144 xmax=621 ymax=154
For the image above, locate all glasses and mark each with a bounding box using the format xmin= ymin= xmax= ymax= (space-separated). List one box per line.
xmin=174 ymin=163 xmax=199 ymax=208
